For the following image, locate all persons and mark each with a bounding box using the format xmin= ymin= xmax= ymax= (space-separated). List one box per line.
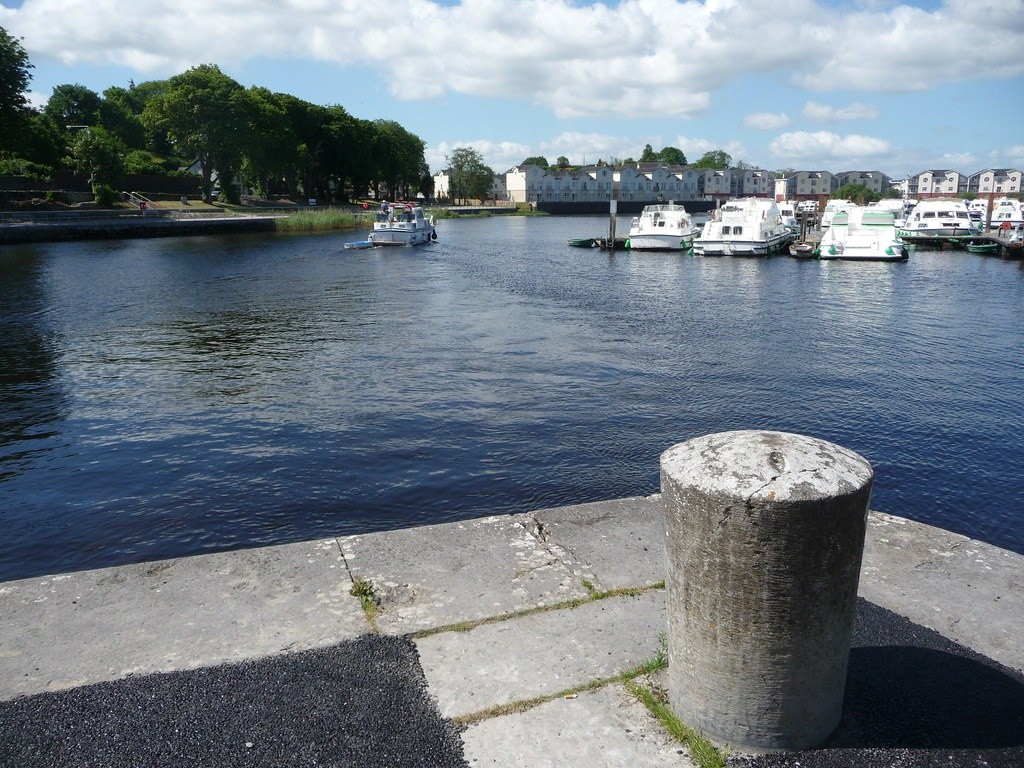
xmin=140 ymin=202 xmax=146 ymax=217
xmin=381 ymin=200 xmax=388 ymax=217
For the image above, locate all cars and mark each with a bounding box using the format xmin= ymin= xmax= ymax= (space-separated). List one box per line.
xmin=211 ymin=191 xmax=221 ymax=200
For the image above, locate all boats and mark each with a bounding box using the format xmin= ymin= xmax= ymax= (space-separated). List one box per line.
xmin=818 ymin=209 xmax=904 ymax=259
xmin=693 ymin=196 xmax=791 ymax=256
xmin=568 ymin=238 xmax=595 ymax=248
xmin=344 ymin=241 xmax=373 ymax=250
xmin=777 ymin=198 xmax=1024 ymax=236
xmin=628 ymin=200 xmax=702 ymax=250
xmin=368 ymin=199 xmax=436 ymax=246
xmin=789 ymin=241 xmax=814 ymax=259
xmin=966 ymin=241 xmax=999 ymax=255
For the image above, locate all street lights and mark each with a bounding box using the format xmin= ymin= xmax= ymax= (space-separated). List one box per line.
xmin=66 ymin=125 xmax=94 ymax=192
